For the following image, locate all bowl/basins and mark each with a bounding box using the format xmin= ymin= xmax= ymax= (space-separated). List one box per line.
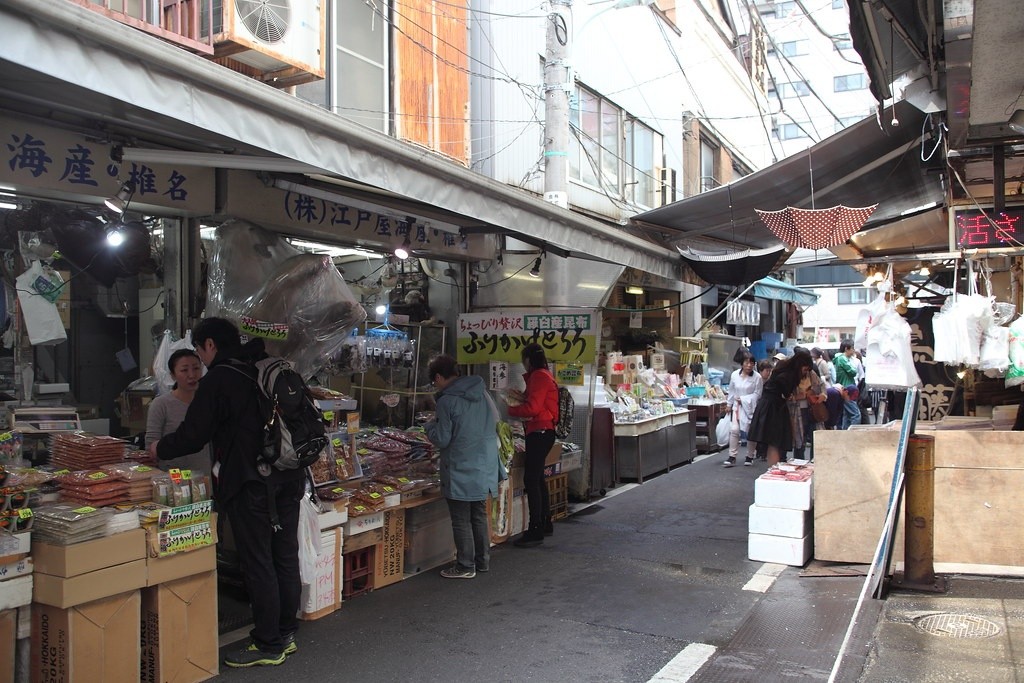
xmin=685 ymin=386 xmax=705 ymax=396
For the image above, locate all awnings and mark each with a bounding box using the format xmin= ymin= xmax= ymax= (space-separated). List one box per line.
xmin=828 ymin=203 xmax=951 ymax=279
xmin=1 ymin=1 xmax=737 ymax=336
xmin=705 ymin=278 xmax=821 ymax=326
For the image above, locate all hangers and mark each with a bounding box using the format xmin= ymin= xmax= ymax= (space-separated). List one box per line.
xmin=364 ymin=304 xmax=409 ymax=341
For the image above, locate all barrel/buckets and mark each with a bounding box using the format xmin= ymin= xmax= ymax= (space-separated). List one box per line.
xmin=748 ymin=341 xmax=767 ymax=362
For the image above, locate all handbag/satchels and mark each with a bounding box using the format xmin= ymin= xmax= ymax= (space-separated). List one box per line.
xmin=482 ymin=389 xmax=514 ymax=473
xmin=808 ymin=401 xmax=829 ymax=423
xmin=857 ymin=378 xmax=872 ymax=409
xmin=841 ymin=385 xmax=859 ymax=402
xmin=715 ymin=412 xmax=731 ymax=447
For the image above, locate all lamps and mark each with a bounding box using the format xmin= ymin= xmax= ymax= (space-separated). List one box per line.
xmin=110 ymin=144 xmax=342 ymax=177
xmin=104 ymin=181 xmax=135 ymax=213
xmin=1008 ymin=110 xmax=1024 ymax=135
xmin=529 ymin=257 xmax=542 ymax=277
xmin=395 ymin=237 xmax=411 ymax=259
xmin=875 ymin=263 xmax=886 ymax=279
xmin=272 ymin=174 xmax=464 ymax=237
xmin=896 ymin=298 xmax=909 ymax=314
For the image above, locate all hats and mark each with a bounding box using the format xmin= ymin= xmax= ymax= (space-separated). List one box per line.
xmin=773 ymin=353 xmax=786 ymax=360
xmin=810 ymin=347 xmax=824 ymax=356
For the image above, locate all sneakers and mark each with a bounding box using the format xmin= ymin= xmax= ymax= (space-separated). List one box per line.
xmin=239 ymin=630 xmax=297 ymax=654
xmin=513 ymin=536 xmax=545 ymax=547
xmin=476 ymin=565 xmax=489 ymax=571
xmin=523 ymin=529 xmax=553 ymax=536
xmin=440 ymin=566 xmax=476 ymax=578
xmin=224 ymin=639 xmax=285 ymax=668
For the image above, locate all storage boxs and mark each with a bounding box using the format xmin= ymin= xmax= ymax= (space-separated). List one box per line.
xmin=54 ymin=271 xmax=74 ymax=300
xmin=747 ymin=461 xmax=814 ymax=567
xmin=613 ymin=410 xmax=690 ymax=437
xmin=298 ymin=432 xmax=584 ymax=609
xmin=55 ymin=300 xmax=71 ymax=329
xmin=0 ymin=511 xmax=221 ymax=683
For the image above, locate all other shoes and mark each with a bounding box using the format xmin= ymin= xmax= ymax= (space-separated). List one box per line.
xmin=744 ymin=457 xmax=754 ymax=465
xmin=724 ymin=456 xmax=736 ymax=466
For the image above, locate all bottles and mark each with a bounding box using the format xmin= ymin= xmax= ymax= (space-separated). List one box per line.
xmin=47 ymin=269 xmax=65 ymax=295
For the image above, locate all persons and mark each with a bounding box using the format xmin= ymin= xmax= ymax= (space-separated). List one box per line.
xmin=146 ymin=349 xmax=213 ymax=498
xmin=747 ymin=351 xmax=813 ymax=466
xmin=789 ymin=339 xmax=871 ymax=460
xmin=723 ymin=353 xmax=763 ymax=465
xmin=503 ymin=343 xmax=560 ymax=544
xmin=152 ymin=317 xmax=308 ymax=666
xmin=730 ymin=346 xmax=787 ymax=459
xmin=424 ymin=356 xmax=508 ymax=578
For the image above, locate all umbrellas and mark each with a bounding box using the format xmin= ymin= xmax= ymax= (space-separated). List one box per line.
xmin=676 ymin=183 xmax=785 ymax=297
xmin=753 ymin=146 xmax=879 ymax=263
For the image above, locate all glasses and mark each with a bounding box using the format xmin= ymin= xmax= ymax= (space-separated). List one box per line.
xmin=191 ymin=343 xmax=208 ymax=356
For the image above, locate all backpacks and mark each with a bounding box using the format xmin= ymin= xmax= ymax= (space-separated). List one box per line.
xmin=215 ymin=357 xmax=330 ymax=482
xmin=534 ymin=370 xmax=574 ymax=439
